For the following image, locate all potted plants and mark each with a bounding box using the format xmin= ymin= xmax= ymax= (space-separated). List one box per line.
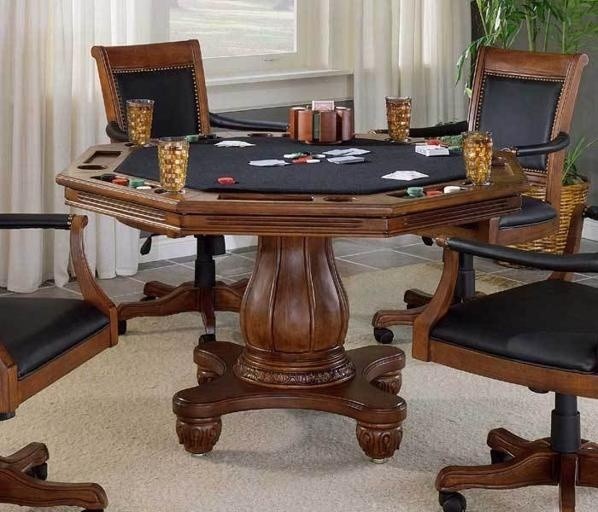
xmin=454 ymin=0 xmax=597 ymax=268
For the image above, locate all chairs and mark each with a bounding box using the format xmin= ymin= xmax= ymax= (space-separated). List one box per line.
xmin=372 ymin=46 xmax=590 ymax=343
xmin=87 ymin=38 xmax=253 ymax=347
xmin=409 ymin=201 xmax=597 ymax=511
xmin=0 ymin=211 xmax=125 ymax=512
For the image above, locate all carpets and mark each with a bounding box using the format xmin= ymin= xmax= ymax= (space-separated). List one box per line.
xmin=0 ymin=263 xmax=597 ymax=510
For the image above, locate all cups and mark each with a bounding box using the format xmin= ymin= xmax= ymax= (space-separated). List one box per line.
xmin=126 ymin=98 xmax=154 ymax=145
xmin=461 ymin=131 xmax=494 ymax=185
xmin=384 ymin=97 xmax=413 ymax=142
xmin=157 ymin=137 xmax=190 ymax=195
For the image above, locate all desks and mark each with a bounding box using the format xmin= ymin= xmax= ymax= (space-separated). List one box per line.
xmin=54 ymin=130 xmax=531 ymax=465
